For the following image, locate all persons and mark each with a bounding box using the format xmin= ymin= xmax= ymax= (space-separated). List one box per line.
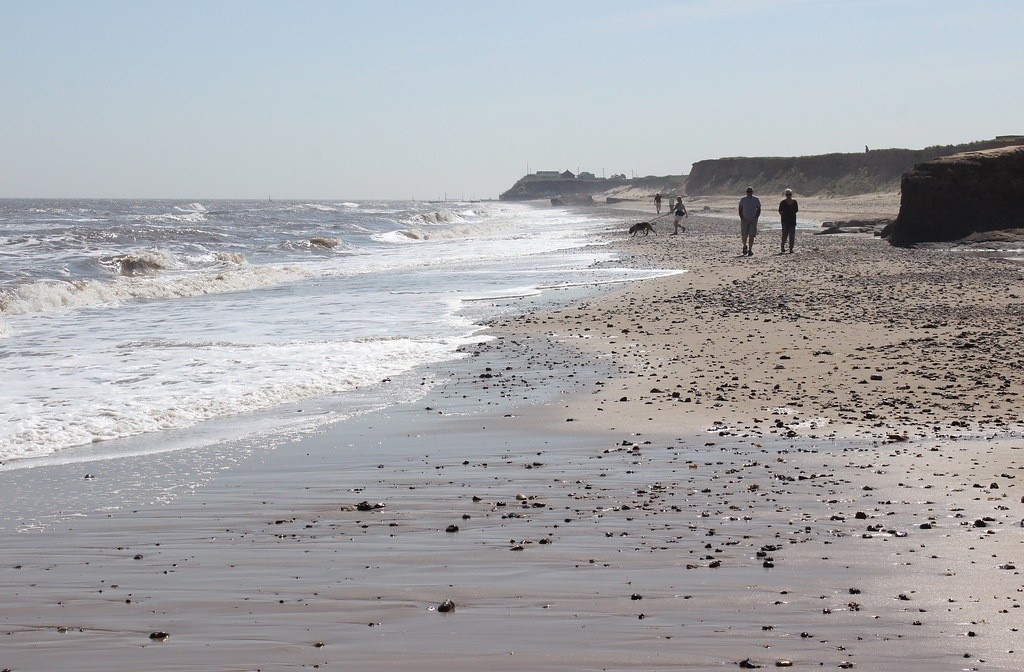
xmin=778 ymin=190 xmax=799 ymax=253
xmin=739 ymin=188 xmax=761 ymax=257
xmin=669 ymin=196 xmax=674 ymax=214
xmin=654 ymin=194 xmax=662 ymax=216
xmin=669 ymin=196 xmax=687 ymax=235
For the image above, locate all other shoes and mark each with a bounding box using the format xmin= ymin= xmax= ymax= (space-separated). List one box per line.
xmin=682 ymin=227 xmax=686 ymax=233
xmin=748 ymin=251 xmax=753 ymax=255
xmin=781 ymin=243 xmax=786 ymax=252
xmin=670 ymin=232 xmax=677 ymax=235
xmin=790 ymin=247 xmax=793 ymax=253
xmin=743 ymin=245 xmax=747 ymax=255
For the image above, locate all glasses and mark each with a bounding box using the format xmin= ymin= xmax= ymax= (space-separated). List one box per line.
xmin=746 ymin=190 xmax=752 ymax=192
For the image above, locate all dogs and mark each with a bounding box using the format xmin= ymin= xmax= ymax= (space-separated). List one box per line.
xmin=629 ymin=222 xmax=657 ymax=237
